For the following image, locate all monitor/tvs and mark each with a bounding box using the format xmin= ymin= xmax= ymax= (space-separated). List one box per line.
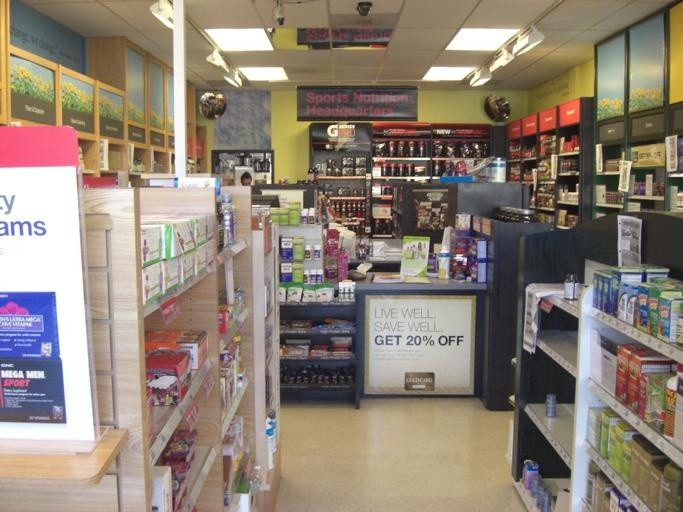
xmin=250 ymin=194 xmax=280 ymax=207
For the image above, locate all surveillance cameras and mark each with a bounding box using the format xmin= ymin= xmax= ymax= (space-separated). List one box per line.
xmin=276 ymin=6 xmax=286 ymax=26
xmin=267 ymin=28 xmax=275 ymax=33
xmin=357 ymin=3 xmax=373 ymax=16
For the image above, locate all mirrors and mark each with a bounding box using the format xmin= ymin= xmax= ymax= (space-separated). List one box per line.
xmin=482 ymin=94 xmax=512 ymax=125
xmin=198 ymin=91 xmax=227 ymax=120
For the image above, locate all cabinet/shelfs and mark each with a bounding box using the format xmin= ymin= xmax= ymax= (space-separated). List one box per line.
xmin=275 ymin=302 xmax=356 ymax=402
xmin=520 ymin=282 xmax=681 ymax=512
xmin=507 ymin=96 xmax=595 ymax=234
xmin=0 ymin=2 xmax=205 ymax=175
xmin=308 ymin=121 xmax=491 ymax=235
xmin=590 ymin=0 xmax=682 ymax=226
xmin=210 ymin=147 xmax=275 ymax=188
xmin=0 ymin=187 xmax=281 ymax=512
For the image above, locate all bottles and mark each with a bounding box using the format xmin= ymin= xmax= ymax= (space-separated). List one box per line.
xmin=543 ymin=390 xmax=558 ymax=420
xmin=374 ymin=141 xmax=491 ymax=180
xmin=222 ymin=192 xmax=237 ymax=246
xmin=373 ymin=184 xmax=406 ymax=236
xmin=518 ymin=456 xmax=553 ymax=511
xmin=323 ymin=182 xmax=365 ymax=220
xmin=563 ymin=273 xmax=580 ymax=299
xmin=265 ymin=409 xmax=277 ymax=472
xmin=300 ymin=208 xmax=324 ymax=284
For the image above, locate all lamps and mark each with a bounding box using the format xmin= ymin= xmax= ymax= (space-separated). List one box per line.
xmin=148 ymin=1 xmax=242 ymax=91
xmin=467 ymin=24 xmax=544 ymax=88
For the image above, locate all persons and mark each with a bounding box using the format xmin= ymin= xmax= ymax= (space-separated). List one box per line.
xmin=241 ymin=172 xmax=252 ymax=186
